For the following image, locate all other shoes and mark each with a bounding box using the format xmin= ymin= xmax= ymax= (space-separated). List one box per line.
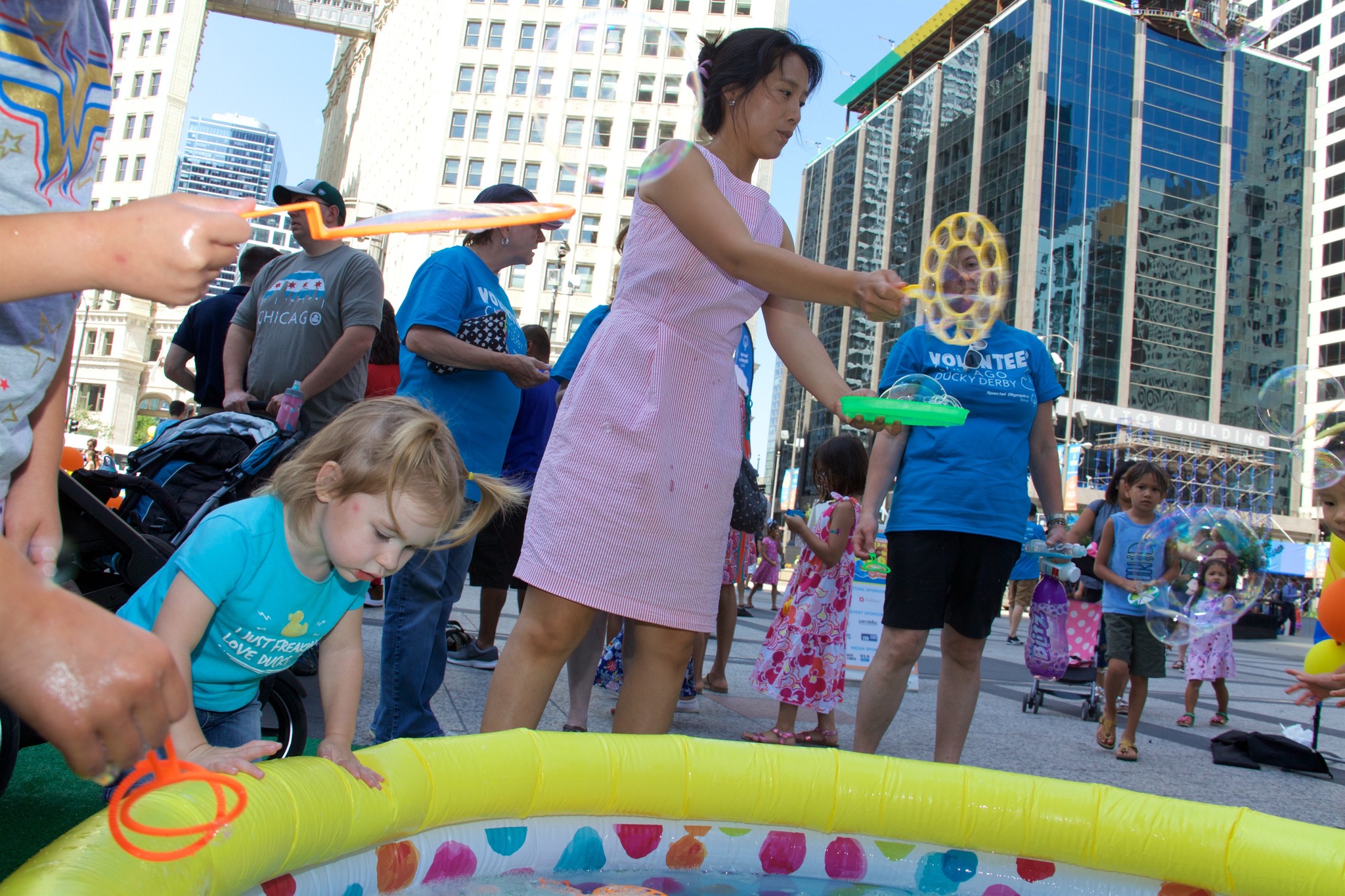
xmin=676 ymin=697 xmax=699 ymax=713
xmin=562 ymin=724 xmax=588 ymax=733
xmin=1172 ymin=660 xmax=1185 ymax=673
xmin=1006 ymin=636 xmax=1024 ymax=646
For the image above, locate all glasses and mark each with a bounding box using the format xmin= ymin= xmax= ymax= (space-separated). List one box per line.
xmin=296 ymin=198 xmax=331 ymax=212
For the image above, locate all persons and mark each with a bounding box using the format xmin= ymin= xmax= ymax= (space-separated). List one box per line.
xmin=481 ymin=25 xmax=905 ymax=737
xmin=1282 ymin=429 xmax=1345 ymax=710
xmin=1167 ymin=515 xmax=1237 ymax=666
xmin=1246 ymin=580 xmax=1323 ymax=637
xmin=153 ymin=400 xmax=186 ymax=439
xmin=222 ymin=179 xmax=383 ymax=674
xmin=0 ymin=0 xmax=254 ymax=793
xmin=737 ymin=521 xmax=783 ymax=611
xmin=165 ymin=246 xmax=284 ymax=416
xmin=1067 ymin=458 xmax=1228 ymax=720
xmin=1093 ymin=462 xmax=1180 ymax=761
xmin=852 ymin=226 xmax=1070 ymax=765
xmin=692 ymin=389 xmax=746 ymax=692
xmin=548 ymin=221 xmax=700 ymax=714
xmin=742 ymin=433 xmax=869 ymax=749
xmin=447 ymin=324 xmax=561 ymax=669
xmin=1166 ymin=559 xmax=1237 ymax=726
xmin=110 ymin=395 xmax=534 ymax=798
xmin=1005 ymin=503 xmax=1047 ymax=645
xmin=368 ymin=182 xmax=563 ymax=743
xmin=80 ymin=438 xmax=114 ymax=470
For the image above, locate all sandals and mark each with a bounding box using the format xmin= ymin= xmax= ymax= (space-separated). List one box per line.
xmin=1209 ymin=711 xmax=1230 ymax=727
xmin=1094 ymin=686 xmax=1105 ymax=700
xmin=796 ymin=726 xmax=839 ymax=747
xmin=741 ymin=727 xmax=796 ymax=746
xmin=1115 ymin=696 xmax=1129 ymax=713
xmin=1177 ymin=712 xmax=1195 ymax=727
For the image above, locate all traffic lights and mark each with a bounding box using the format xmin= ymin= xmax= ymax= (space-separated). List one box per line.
xmin=69 ymin=420 xmax=78 ymax=434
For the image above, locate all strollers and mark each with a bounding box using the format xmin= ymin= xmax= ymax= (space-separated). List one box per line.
xmin=58 ymin=397 xmax=312 ymax=761
xmin=1023 ymin=574 xmax=1101 ymax=722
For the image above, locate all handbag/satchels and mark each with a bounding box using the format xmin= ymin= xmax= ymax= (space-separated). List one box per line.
xmin=593 ymin=626 xmax=696 ymax=699
xmin=445 ymin=620 xmax=477 ymax=652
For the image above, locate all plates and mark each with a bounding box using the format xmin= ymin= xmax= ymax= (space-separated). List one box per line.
xmin=839 ymin=395 xmax=971 ymax=427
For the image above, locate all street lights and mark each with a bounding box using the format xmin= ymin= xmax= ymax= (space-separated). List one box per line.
xmin=65 ymin=295 xmax=117 ymax=433
xmin=1036 ymin=334 xmax=1078 ymax=505
xmin=546 ymin=239 xmax=571 ymax=341
xmin=782 ymin=395 xmax=819 ymax=562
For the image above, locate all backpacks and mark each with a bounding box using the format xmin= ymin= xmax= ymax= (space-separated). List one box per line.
xmin=730 ymin=454 xmax=768 ymax=536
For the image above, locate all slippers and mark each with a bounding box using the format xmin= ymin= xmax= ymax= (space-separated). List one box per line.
xmin=700 ymin=673 xmax=728 ymax=693
xmin=1116 ymin=739 xmax=1139 ymax=761
xmin=1096 ymin=715 xmax=1117 ymax=749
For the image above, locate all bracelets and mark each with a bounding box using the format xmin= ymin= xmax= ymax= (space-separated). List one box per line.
xmin=1043 ymin=513 xmax=1067 ymax=534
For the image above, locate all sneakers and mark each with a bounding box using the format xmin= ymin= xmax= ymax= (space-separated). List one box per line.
xmin=362 ymin=583 xmax=385 ymax=608
xmin=447 ymin=641 xmax=498 ymax=669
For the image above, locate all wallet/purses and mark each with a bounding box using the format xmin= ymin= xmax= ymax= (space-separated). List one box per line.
xmin=427 ymin=309 xmax=509 ymax=375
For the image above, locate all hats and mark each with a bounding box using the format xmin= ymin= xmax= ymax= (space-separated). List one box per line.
xmin=474 ymin=183 xmax=565 ymax=234
xmin=272 ymin=177 xmax=348 ymax=220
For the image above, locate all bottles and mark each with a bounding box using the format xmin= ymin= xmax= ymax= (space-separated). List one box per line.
xmin=1038 ymin=556 xmax=1081 ymax=583
xmin=276 ymin=380 xmax=304 ymax=437
xmin=1024 ymin=539 xmax=1087 ymax=559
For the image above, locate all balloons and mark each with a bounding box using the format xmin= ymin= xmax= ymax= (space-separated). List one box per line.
xmin=1330 ymin=533 xmax=1345 ymax=572
xmin=1316 ymin=577 xmax=1345 ymax=645
xmin=1304 ymin=639 xmax=1345 ymax=674
